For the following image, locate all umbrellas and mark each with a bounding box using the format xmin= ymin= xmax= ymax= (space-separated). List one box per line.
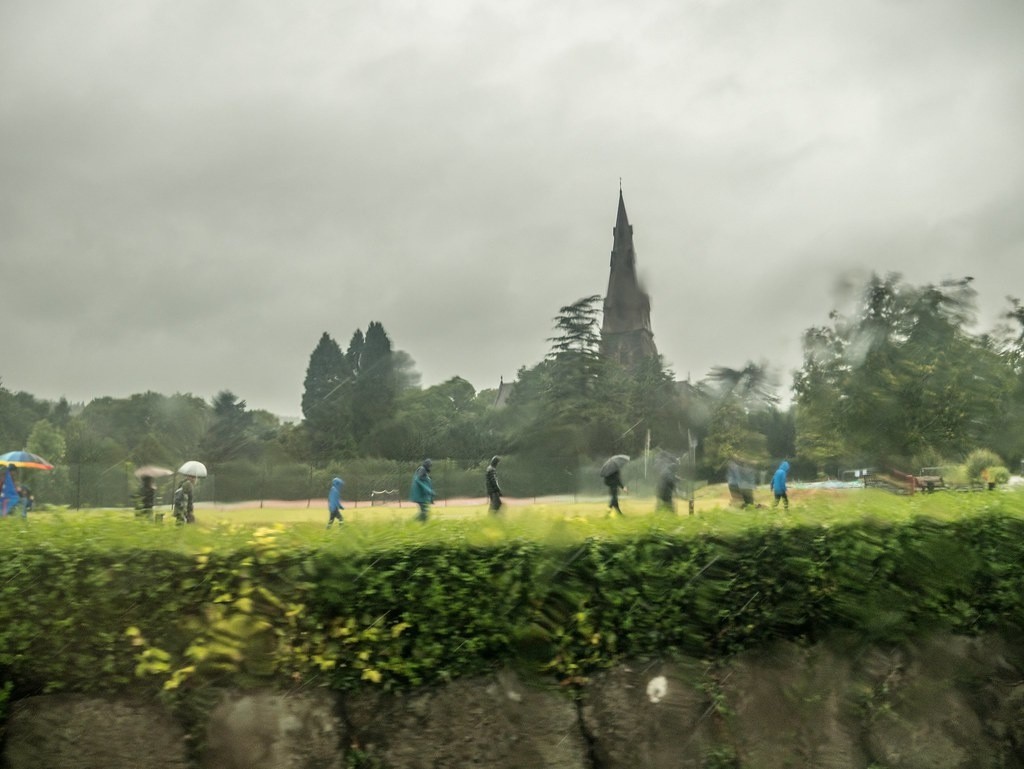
xmin=600 ymin=453 xmax=631 ymax=476
xmin=133 ymin=464 xmax=173 ymax=480
xmin=178 ymin=460 xmax=207 ymax=478
xmin=0 ymin=450 xmax=54 ymax=470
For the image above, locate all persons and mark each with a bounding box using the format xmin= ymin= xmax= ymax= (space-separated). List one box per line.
xmin=0 ymin=463 xmax=34 ymax=518
xmin=769 ymin=462 xmax=791 ymax=508
xmin=604 ymin=472 xmax=626 ymax=518
xmin=326 ymin=478 xmax=344 ymax=530
xmin=654 ymin=465 xmax=680 ymax=516
xmin=486 ymin=456 xmax=504 ymax=512
xmin=411 ymin=459 xmax=440 ymax=521
xmin=139 ymin=476 xmax=157 ymax=519
xmin=175 ymin=476 xmax=197 ymax=524
xmin=728 ymin=455 xmax=762 ymax=510
